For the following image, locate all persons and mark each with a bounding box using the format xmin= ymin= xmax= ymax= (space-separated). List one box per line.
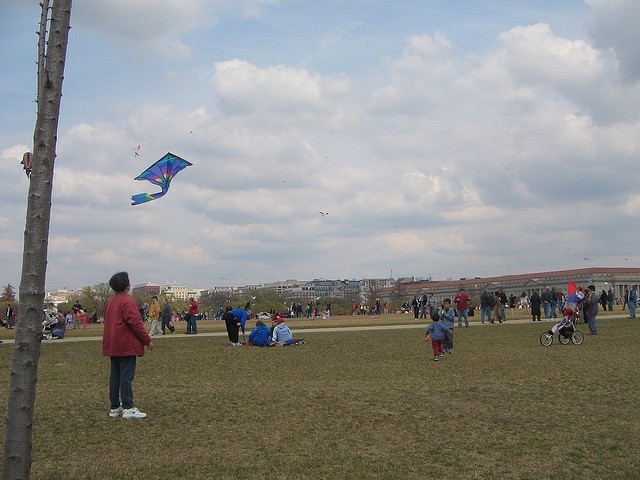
xmin=453 ymin=288 xmax=471 ymax=329
xmin=352 ymin=293 xmax=438 ymax=320
xmin=138 ymin=299 xmax=151 ymax=323
xmin=147 ymin=294 xmax=164 ymax=337
xmin=158 ymin=297 xmax=177 ymax=335
xmin=491 ymin=291 xmax=503 ymax=324
xmin=102 ymin=270 xmax=154 ymax=420
xmin=479 ymin=288 xmax=496 ymax=325
xmin=269 ymin=300 xmax=333 ymax=319
xmin=241 ymin=319 xmax=270 ymax=347
xmin=171 ymin=308 xmax=184 ymax=321
xmin=224 ymin=309 xmax=255 ymax=348
xmin=5 ymin=303 xmax=15 ymax=329
xmin=42 ymin=298 xmax=89 ymax=339
xmin=509 ymin=280 xmax=589 ymax=322
xmin=584 ymin=283 xmax=599 ymax=335
xmin=268 ymin=316 xmax=293 ymax=344
xmin=438 ymin=298 xmax=455 ymax=358
xmin=497 ymin=288 xmax=509 ymax=322
xmin=425 ymin=313 xmax=453 ymax=361
xmin=200 ymin=305 xmax=233 ymax=320
xmin=183 ymin=296 xmax=200 ymax=335
xmin=599 ymin=282 xmax=640 ymax=318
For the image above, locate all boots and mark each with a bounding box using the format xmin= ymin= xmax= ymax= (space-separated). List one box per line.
xmin=491 ymin=319 xmax=495 ymax=324
xmin=498 ymin=315 xmax=502 ymax=323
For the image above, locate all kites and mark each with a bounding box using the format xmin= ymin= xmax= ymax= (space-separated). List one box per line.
xmin=130 ymin=152 xmax=194 ymax=206
xmin=19 ymin=150 xmax=34 ymax=179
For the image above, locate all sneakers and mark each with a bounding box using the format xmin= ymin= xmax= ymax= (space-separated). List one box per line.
xmin=434 ymin=356 xmax=439 ymax=361
xmin=443 ymin=349 xmax=446 ymax=354
xmin=158 ymin=333 xmax=162 ymax=336
xmin=191 ymin=332 xmax=197 ymax=334
xmin=109 ymin=404 xmax=122 ymax=417
xmin=172 ymin=331 xmax=175 ymax=334
xmin=586 ymin=332 xmax=597 ymax=335
xmin=185 ymin=332 xmax=191 ymax=334
xmin=231 ymin=341 xmax=235 ymax=346
xmin=122 ymin=407 xmax=147 ymax=418
xmin=439 ymin=353 xmax=443 ymax=357
xmin=148 ymin=333 xmax=154 ymax=336
xmin=236 ymin=343 xmax=242 ymax=347
xmin=448 ymin=349 xmax=453 ymax=354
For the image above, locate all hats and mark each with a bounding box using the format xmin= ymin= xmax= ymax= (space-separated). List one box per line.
xmin=274 ymin=318 xmax=284 ymax=322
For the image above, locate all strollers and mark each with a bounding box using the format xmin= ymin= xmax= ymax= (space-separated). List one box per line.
xmin=539 ymin=314 xmax=584 ymax=346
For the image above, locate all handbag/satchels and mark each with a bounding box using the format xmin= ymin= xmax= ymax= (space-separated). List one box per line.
xmin=184 ymin=313 xmax=190 ymax=321
